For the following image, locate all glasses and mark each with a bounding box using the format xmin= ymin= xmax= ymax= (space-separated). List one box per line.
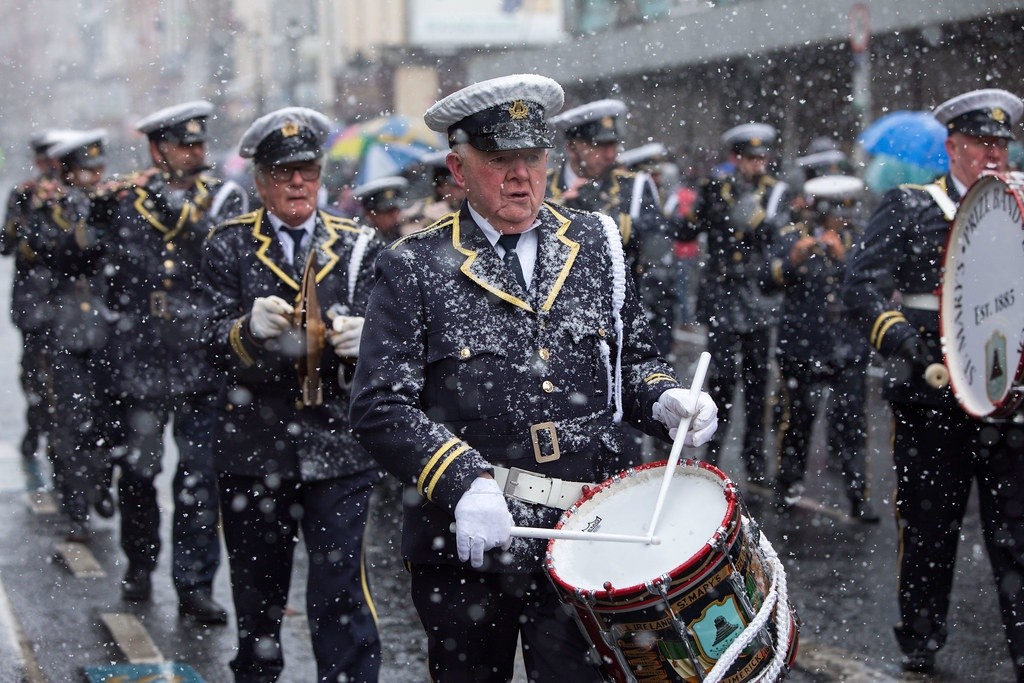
xmin=261 ymin=164 xmax=320 ymax=183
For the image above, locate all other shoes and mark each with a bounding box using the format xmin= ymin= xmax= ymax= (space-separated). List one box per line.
xmin=19 ymin=422 xmax=941 ymax=683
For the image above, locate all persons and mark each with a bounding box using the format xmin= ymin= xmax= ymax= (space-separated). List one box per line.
xmin=2 ymin=125 xmax=143 ymax=547
xmin=188 ymin=106 xmax=393 ymax=682
xmin=323 ymin=100 xmax=877 ymax=524
xmin=346 ymin=73 xmax=719 ymax=683
xmin=845 ymin=88 xmax=1022 ymax=683
xmin=88 ymin=99 xmax=248 ymax=624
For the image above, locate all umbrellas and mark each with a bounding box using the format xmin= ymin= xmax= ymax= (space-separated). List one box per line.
xmin=856 ymin=108 xmax=952 ymax=174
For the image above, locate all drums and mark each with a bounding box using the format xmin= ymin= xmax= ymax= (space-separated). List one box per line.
xmin=937 ymin=170 xmax=1024 ymax=419
xmin=540 ymin=458 xmax=802 ymax=682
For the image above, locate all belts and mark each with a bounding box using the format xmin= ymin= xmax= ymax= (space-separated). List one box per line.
xmin=898 ymin=292 xmax=942 ymax=311
xmin=488 ymin=465 xmax=600 ymax=511
xmin=461 ymin=408 xmax=628 ymax=464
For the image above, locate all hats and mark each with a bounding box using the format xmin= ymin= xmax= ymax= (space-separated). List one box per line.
xmin=803 ymin=175 xmax=864 ymax=208
xmin=933 ymin=88 xmax=1024 ymax=140
xmin=550 ymin=99 xmax=628 ymax=143
xmin=46 ymin=128 xmax=110 ymax=167
xmin=795 ymin=149 xmax=847 ymax=174
xmin=415 ymin=152 xmax=460 ymax=188
xmin=33 ymin=128 xmax=71 ymax=158
xmin=352 ymin=176 xmax=409 ymax=215
xmin=136 ymin=100 xmax=214 ymax=146
xmin=722 ymin=123 xmax=776 ymax=158
xmin=239 ymin=106 xmax=337 ymax=167
xmin=424 ymin=74 xmax=564 ymax=151
xmin=615 ymin=142 xmax=669 ymax=170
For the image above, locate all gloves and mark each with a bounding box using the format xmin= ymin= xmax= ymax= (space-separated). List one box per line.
xmin=330 ymin=315 xmax=365 ymax=356
xmin=454 ymin=477 xmax=515 ymax=567
xmin=249 ymin=295 xmax=295 ymax=338
xmin=895 ymin=334 xmax=935 ymax=374
xmin=652 ymin=387 xmax=720 ymax=447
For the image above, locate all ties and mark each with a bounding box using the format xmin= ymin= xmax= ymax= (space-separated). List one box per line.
xmin=278 ymin=226 xmax=307 ymax=279
xmin=497 ymin=233 xmax=527 ymax=292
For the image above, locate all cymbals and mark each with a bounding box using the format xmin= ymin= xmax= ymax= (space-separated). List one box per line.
xmin=282 ymin=249 xmax=339 ymax=410
